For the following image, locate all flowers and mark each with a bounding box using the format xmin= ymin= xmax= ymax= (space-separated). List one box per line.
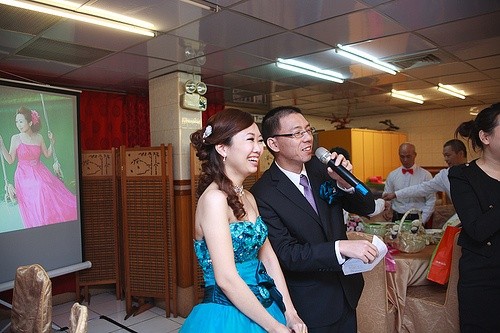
xmin=366 ymin=174 xmax=383 ymax=186
xmin=31 ymin=110 xmax=41 ymax=126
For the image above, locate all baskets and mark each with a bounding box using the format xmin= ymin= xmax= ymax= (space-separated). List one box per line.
xmin=384 ymin=208 xmax=426 ymax=253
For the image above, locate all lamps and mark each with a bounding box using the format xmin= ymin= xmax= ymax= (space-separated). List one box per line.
xmin=436 ymin=82 xmax=466 ymax=101
xmin=179 ymin=80 xmax=207 ymax=111
xmin=184 ymin=44 xmax=207 ymax=67
xmin=179 ymin=0 xmax=221 ymax=14
xmin=0 ymin=0 xmax=158 ymax=38
xmin=391 ymin=89 xmax=426 ymax=104
xmin=335 ymin=47 xmax=398 ymax=76
xmin=275 ymin=62 xmax=344 ymax=84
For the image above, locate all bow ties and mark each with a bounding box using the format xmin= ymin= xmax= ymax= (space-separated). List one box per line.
xmin=402 ymin=167 xmax=413 ymax=175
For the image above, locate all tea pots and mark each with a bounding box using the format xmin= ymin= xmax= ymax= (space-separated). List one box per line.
xmin=393 ymin=208 xmax=429 ymax=254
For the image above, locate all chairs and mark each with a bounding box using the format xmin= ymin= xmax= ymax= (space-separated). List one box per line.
xmin=346 ymin=231 xmax=399 ymax=333
xmin=70 ymin=303 xmax=89 ymax=333
xmin=403 ymin=228 xmax=462 ymax=333
xmin=13 ymin=264 xmax=52 ymax=333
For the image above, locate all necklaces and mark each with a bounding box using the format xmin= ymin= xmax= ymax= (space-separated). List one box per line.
xmin=234 ymin=184 xmax=246 ymax=197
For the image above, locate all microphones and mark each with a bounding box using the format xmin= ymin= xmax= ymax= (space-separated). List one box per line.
xmin=315 ymin=147 xmax=368 ymax=196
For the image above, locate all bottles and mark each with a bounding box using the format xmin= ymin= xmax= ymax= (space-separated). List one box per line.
xmin=346 ymin=213 xmax=364 ymax=232
xmin=395 ymin=220 xmax=412 ymax=231
xmin=365 ymin=223 xmax=387 ymax=238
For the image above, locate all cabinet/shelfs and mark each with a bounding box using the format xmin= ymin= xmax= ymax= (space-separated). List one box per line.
xmin=317 ymin=129 xmax=407 ymax=184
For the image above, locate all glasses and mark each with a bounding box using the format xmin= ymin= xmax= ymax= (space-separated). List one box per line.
xmin=266 ymin=128 xmax=312 ymax=138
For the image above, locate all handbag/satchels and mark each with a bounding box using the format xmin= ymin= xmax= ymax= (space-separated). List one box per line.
xmin=426 ymin=224 xmax=462 ymax=285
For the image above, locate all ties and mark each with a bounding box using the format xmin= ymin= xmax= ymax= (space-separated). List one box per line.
xmin=299 ymin=174 xmax=319 ymax=218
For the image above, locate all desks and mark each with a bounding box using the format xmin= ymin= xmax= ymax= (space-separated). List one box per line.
xmin=347 ymin=219 xmax=454 ymax=310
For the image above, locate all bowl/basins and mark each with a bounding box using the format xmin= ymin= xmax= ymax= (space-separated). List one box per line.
xmin=425 ymin=229 xmax=442 ymax=241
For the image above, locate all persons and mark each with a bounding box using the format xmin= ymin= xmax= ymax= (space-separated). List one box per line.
xmin=448 ymin=102 xmax=500 ymax=333
xmin=329 ymin=143 xmax=436 ymax=232
xmin=0 ymin=107 xmax=77 ymax=229
xmin=381 ymin=139 xmax=467 ymax=203
xmin=248 ymin=105 xmax=379 ymax=333
xmin=177 ymin=108 xmax=308 ymax=333
